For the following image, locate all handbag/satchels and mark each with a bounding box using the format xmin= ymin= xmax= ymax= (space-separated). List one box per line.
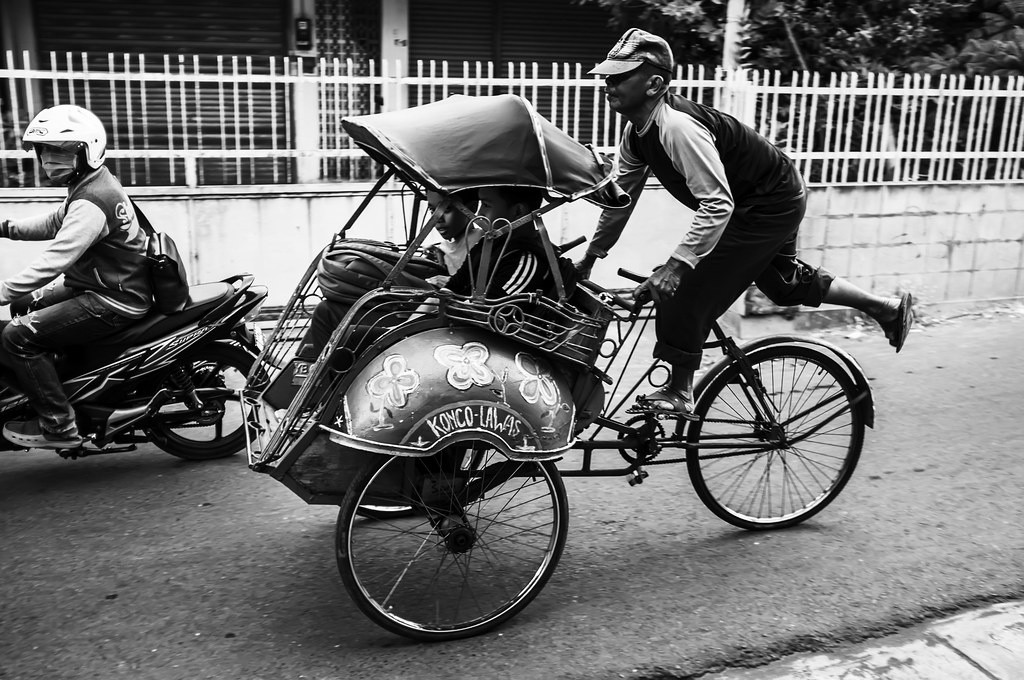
xmin=148 ymin=229 xmax=188 ymax=313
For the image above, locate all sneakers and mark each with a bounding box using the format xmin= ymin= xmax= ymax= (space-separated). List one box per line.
xmin=4 ymin=418 xmax=82 ymax=449
xmin=276 ymin=408 xmax=318 ymax=430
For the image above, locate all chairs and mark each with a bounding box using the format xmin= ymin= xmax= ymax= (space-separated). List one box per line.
xmin=318 ymin=254 xmax=581 ymax=408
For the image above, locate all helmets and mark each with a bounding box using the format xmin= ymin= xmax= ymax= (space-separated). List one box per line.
xmin=22 ymin=104 xmax=107 ymax=168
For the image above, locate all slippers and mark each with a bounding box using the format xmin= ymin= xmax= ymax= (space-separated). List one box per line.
xmin=628 ymin=386 xmax=701 ymax=421
xmin=883 ymin=294 xmax=917 ymax=352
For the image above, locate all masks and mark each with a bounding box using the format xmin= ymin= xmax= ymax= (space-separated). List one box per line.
xmin=39 ymin=152 xmax=79 ymax=181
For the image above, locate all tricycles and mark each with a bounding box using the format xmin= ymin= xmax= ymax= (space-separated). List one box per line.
xmin=234 ymin=90 xmax=876 ymax=645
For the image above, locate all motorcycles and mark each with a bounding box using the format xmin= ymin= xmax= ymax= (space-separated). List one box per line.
xmin=0 ymin=270 xmax=291 ymax=465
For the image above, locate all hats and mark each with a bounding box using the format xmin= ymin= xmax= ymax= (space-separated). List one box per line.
xmin=587 ymin=26 xmax=676 ymax=76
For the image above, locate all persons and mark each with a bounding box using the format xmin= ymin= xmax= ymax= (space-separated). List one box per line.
xmin=377 ymin=166 xmax=550 ymax=356
xmin=1 ymin=104 xmax=152 ymax=449
xmin=274 ymin=171 xmax=483 ymax=423
xmin=572 ymin=26 xmax=912 ymax=421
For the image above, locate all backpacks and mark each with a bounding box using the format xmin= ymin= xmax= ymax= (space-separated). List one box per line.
xmin=317 ymin=231 xmax=448 ymax=309
xmin=491 ymin=241 xmax=579 ymax=332
